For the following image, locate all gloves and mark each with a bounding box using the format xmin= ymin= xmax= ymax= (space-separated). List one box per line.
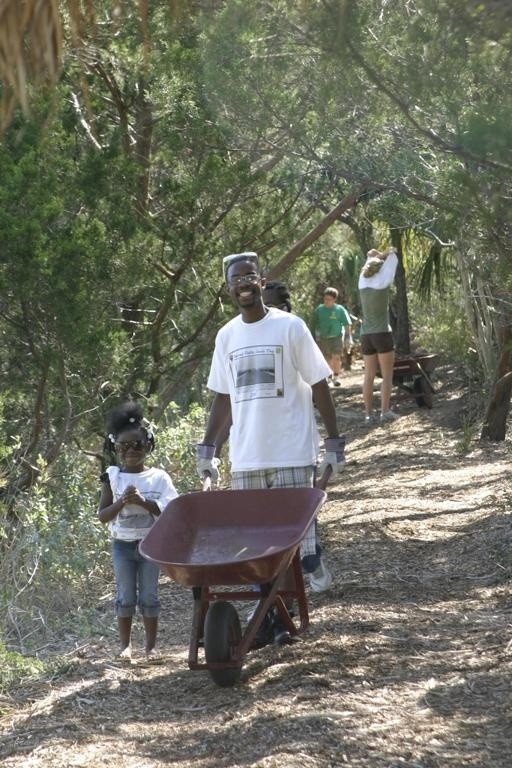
xmin=344 ymin=335 xmax=352 ymax=345
xmin=195 ymin=443 xmax=222 ymax=483
xmin=321 ymin=437 xmax=347 ymax=475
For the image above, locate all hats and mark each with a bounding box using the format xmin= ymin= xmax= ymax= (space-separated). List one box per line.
xmin=222 ymin=251 xmax=259 ymax=282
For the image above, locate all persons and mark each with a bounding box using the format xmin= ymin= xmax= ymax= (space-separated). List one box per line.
xmin=196 ymin=250 xmax=347 ymax=643
xmin=97 ymin=403 xmax=179 ymax=660
xmin=340 ymin=299 xmax=363 ymax=371
xmin=357 ymin=246 xmax=400 ymax=424
xmin=261 ymin=280 xmax=292 ymax=313
xmin=309 ymin=286 xmax=353 ymax=387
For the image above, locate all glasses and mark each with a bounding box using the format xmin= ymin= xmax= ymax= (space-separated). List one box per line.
xmin=230 ymin=274 xmax=260 ymax=284
xmin=114 ymin=440 xmax=148 ymax=452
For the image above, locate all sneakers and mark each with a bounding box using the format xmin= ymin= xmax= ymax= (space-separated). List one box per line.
xmin=380 ymin=410 xmax=400 ymax=421
xmin=365 ymin=415 xmax=378 ymax=423
xmin=308 ymin=557 xmax=332 ymax=594
xmin=330 ymin=377 xmax=341 ymax=387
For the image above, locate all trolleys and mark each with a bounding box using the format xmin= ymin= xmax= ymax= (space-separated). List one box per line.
xmin=138 ymin=465 xmax=333 ymax=686
xmin=364 ymin=352 xmax=441 ymax=410
